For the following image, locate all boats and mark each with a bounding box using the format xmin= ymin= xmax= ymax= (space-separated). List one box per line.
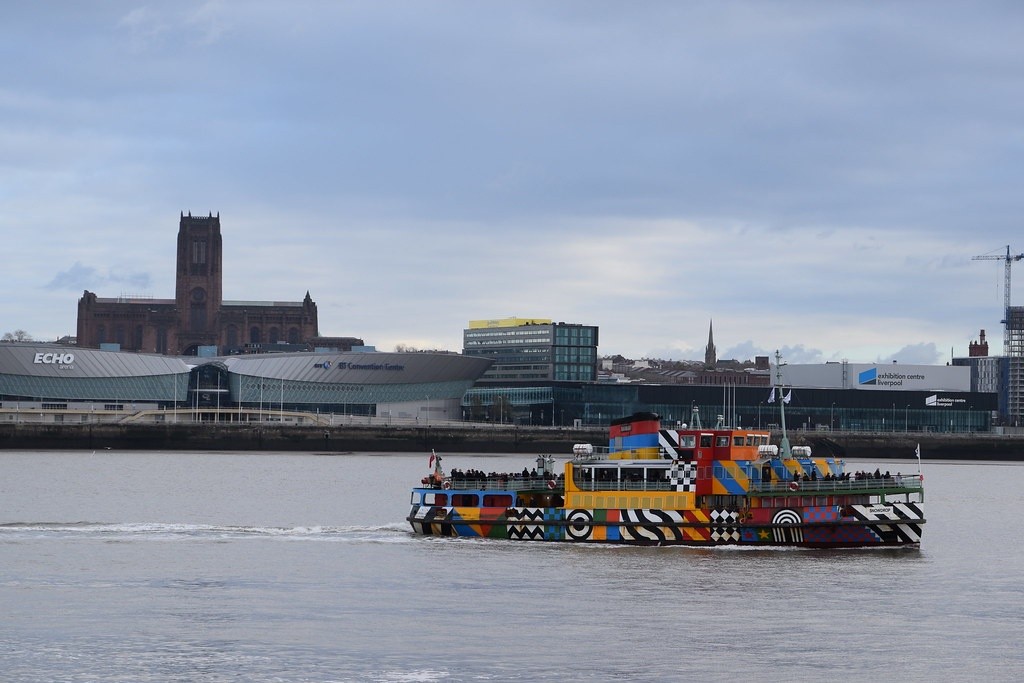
xmin=407 ymin=402 xmax=927 ymax=551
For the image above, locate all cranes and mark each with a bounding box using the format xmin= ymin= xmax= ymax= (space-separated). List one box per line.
xmin=968 ymin=243 xmax=1024 ymax=357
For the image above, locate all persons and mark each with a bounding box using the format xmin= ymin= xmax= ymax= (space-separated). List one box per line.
xmin=874 ymin=468 xmax=890 ymax=480
xmin=531 ymin=468 xmax=537 ymax=479
xmin=841 ymin=472 xmax=850 ymax=480
xmin=451 ymin=468 xmax=521 ymax=482
xmin=855 ymin=470 xmax=872 ymax=479
xmin=803 ymin=473 xmax=809 ymax=481
xmin=896 ymin=472 xmax=905 ymax=488
xmin=543 ymin=468 xmax=552 ymax=480
xmin=812 ymin=468 xmax=817 ymax=481
xmin=794 ymin=470 xmax=799 ymax=481
xmin=831 ymin=474 xmax=837 ymax=481
xmin=763 ymin=469 xmax=770 ymax=480
xmin=522 ymin=467 xmax=530 ymax=480
xmin=825 ymin=473 xmax=831 ymax=480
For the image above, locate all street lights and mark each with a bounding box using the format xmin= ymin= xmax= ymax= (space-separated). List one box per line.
xmin=830 ymin=402 xmax=836 ymax=432
xmin=550 ymin=396 xmax=555 ymax=426
xmin=892 ymin=403 xmax=896 ymax=432
xmin=905 ymin=404 xmax=910 ymax=434
xmin=968 ymin=405 xmax=973 ymax=433
xmin=758 ymin=401 xmax=764 ymax=431
xmin=425 ymin=394 xmax=430 ymax=425
xmin=498 ymin=395 xmax=503 ymax=425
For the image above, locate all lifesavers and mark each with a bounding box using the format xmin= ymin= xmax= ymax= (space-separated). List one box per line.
xmin=548 ymin=480 xmax=556 ymax=490
xmin=789 ymin=482 xmax=799 ymax=492
xmin=443 ymin=481 xmax=451 ymax=490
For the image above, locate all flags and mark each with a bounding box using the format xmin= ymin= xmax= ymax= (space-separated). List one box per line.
xmin=784 ymin=389 xmax=791 ymax=403
xmin=768 ymin=388 xmax=775 ymax=403
xmin=428 ymin=453 xmax=435 ymax=468
xmin=915 ymin=445 xmax=920 ymax=457
